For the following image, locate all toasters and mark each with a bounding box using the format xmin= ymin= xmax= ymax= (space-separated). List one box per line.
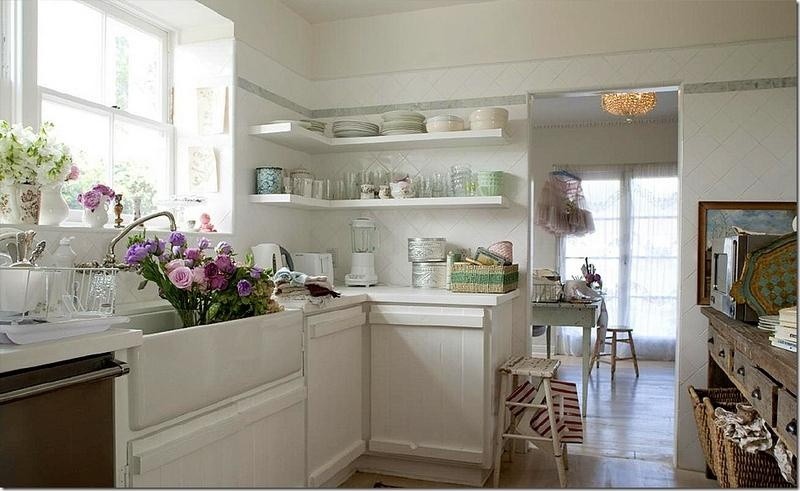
xmin=296 ymin=251 xmax=335 ymax=288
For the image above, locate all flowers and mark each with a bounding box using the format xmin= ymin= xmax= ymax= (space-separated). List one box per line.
xmin=0 ymin=114 xmax=80 ymax=189
xmin=76 ymin=183 xmax=118 ymax=213
xmin=126 ymin=229 xmax=285 ymax=327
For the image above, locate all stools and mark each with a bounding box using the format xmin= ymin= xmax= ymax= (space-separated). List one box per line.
xmin=588 ymin=325 xmax=643 ymax=383
xmin=495 ymin=356 xmax=576 ymax=490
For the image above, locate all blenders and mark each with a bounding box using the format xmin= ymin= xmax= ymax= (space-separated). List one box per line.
xmin=345 ymin=216 xmax=380 ymax=286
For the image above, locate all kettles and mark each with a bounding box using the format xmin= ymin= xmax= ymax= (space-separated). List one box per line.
xmin=252 ymin=243 xmax=296 ymax=280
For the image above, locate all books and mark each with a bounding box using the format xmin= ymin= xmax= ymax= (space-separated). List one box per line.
xmin=768 ymin=306 xmax=797 ymax=355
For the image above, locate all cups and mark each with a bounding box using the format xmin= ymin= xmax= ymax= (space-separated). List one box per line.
xmin=22 ymin=271 xmax=50 ymax=324
xmin=254 ymin=165 xmax=388 ymax=199
xmin=418 ymin=160 xmax=473 ymax=197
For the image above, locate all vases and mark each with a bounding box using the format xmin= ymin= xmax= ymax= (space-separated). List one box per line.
xmin=82 ymin=201 xmax=110 ymax=228
xmin=14 ymin=182 xmax=72 ymax=227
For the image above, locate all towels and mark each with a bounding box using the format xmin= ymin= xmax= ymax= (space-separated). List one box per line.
xmin=271 ymin=265 xmax=340 ymax=308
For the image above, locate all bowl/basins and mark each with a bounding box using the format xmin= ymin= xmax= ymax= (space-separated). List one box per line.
xmin=425 ymin=118 xmax=462 ymax=130
xmin=471 ymin=108 xmax=507 ymax=120
xmin=390 ymin=179 xmax=414 ymax=198
xmin=470 ymin=117 xmax=509 ymax=130
xmin=475 ymin=169 xmax=503 ymax=194
xmin=428 ymin=114 xmax=463 ymax=121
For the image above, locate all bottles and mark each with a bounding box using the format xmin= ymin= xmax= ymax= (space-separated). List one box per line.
xmin=53 ymin=233 xmax=76 ymax=318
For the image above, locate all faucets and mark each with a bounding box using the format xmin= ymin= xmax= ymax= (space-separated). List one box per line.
xmin=101 ymin=210 xmax=176 ymax=269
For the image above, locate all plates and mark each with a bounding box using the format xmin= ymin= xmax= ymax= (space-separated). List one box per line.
xmin=382 ymin=113 xmax=423 ymax=133
xmin=758 ymin=315 xmax=782 ymax=331
xmin=334 ymin=119 xmax=380 ymax=136
xmin=275 ymin=117 xmax=327 ymax=132
xmin=1 ymin=227 xmax=50 ymax=267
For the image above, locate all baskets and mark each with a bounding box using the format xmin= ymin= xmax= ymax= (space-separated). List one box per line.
xmin=725 ymin=429 xmax=799 ymax=488
xmin=704 ymin=398 xmax=749 ymax=489
xmin=687 ymin=384 xmax=748 ymax=476
xmin=0 ymin=267 xmax=117 ymax=324
xmin=451 ymin=265 xmax=518 ymax=293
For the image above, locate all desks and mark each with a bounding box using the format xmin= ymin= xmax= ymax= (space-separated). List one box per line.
xmin=528 ymin=298 xmax=606 ymax=421
xmin=690 ymin=306 xmax=798 ymax=488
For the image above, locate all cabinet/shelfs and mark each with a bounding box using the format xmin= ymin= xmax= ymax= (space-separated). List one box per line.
xmin=247 ymin=121 xmax=511 ymax=208
xmin=304 ymin=306 xmax=367 ymax=490
xmin=365 ymin=306 xmax=490 ymax=463
xmin=123 ymin=379 xmax=306 ymax=491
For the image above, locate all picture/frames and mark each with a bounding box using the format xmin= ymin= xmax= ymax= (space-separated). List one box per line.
xmin=696 ymin=199 xmax=799 ymax=307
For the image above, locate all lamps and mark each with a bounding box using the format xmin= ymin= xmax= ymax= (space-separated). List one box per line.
xmin=596 ymin=92 xmax=659 ymax=123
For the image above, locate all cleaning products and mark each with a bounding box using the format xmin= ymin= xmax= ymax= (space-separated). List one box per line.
xmin=53 ymin=236 xmax=74 ymax=305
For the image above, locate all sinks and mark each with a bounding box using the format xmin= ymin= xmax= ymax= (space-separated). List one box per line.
xmin=109 ymin=310 xmax=223 ymax=335
